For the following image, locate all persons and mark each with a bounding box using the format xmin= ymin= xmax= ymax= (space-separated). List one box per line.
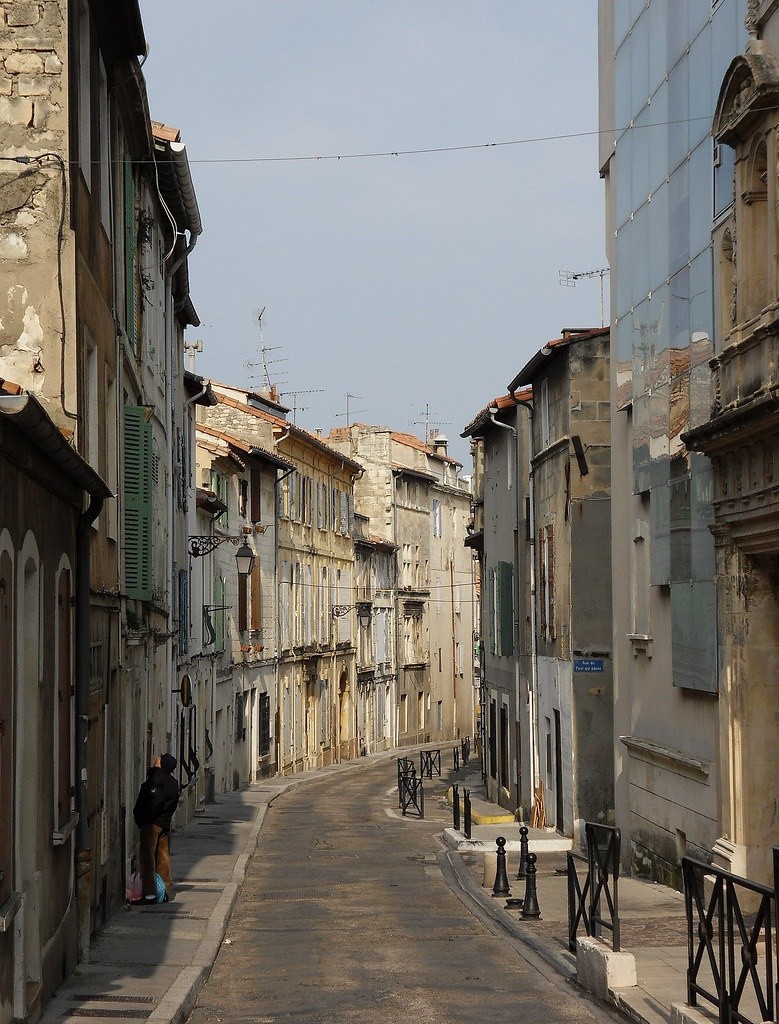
xmin=129 ymin=752 xmax=179 ymax=906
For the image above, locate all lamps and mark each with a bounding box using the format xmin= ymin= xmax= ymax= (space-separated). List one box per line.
xmin=188 ymin=535 xmax=255 ymax=581
xmin=332 ymin=602 xmax=371 ymax=630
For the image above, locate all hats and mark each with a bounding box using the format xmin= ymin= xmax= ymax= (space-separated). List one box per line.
xmin=161 ymin=753 xmax=177 ymax=774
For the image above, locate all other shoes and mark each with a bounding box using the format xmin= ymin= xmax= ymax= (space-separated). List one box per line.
xmin=130 ymin=895 xmax=157 ymax=905
xmin=162 ymin=893 xmax=169 ymax=903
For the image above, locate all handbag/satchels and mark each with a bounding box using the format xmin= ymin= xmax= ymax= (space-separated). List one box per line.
xmin=126 ymin=871 xmax=144 ymax=902
xmin=154 ymin=872 xmax=166 ymax=903
